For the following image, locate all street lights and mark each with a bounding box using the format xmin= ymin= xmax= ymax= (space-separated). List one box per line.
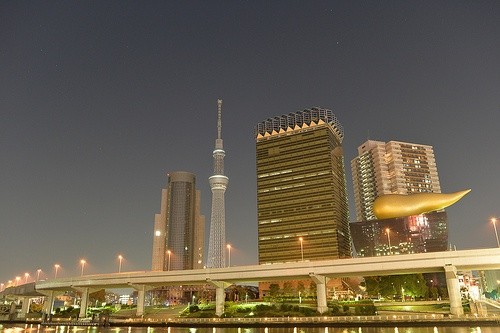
xmin=226 ymin=244 xmax=231 ymax=267
xmin=385 ymin=228 xmax=391 ymax=256
xmin=54 ymin=264 xmax=59 ymax=279
xmin=16 ymin=277 xmax=20 ymax=286
xmin=491 ymin=218 xmax=500 ymax=248
xmin=37 ymin=268 xmax=41 ymax=283
xmin=25 ymin=272 xmax=30 ymax=283
xmin=1 ymin=283 xmax=4 ymax=290
xmin=298 ymin=237 xmax=304 ymax=261
xmin=81 ymin=259 xmax=86 ymax=276
xmin=168 ymin=250 xmax=171 ymax=271
xmin=118 ymin=256 xmax=123 ymax=272
xmin=8 ymin=280 xmax=12 ymax=286
